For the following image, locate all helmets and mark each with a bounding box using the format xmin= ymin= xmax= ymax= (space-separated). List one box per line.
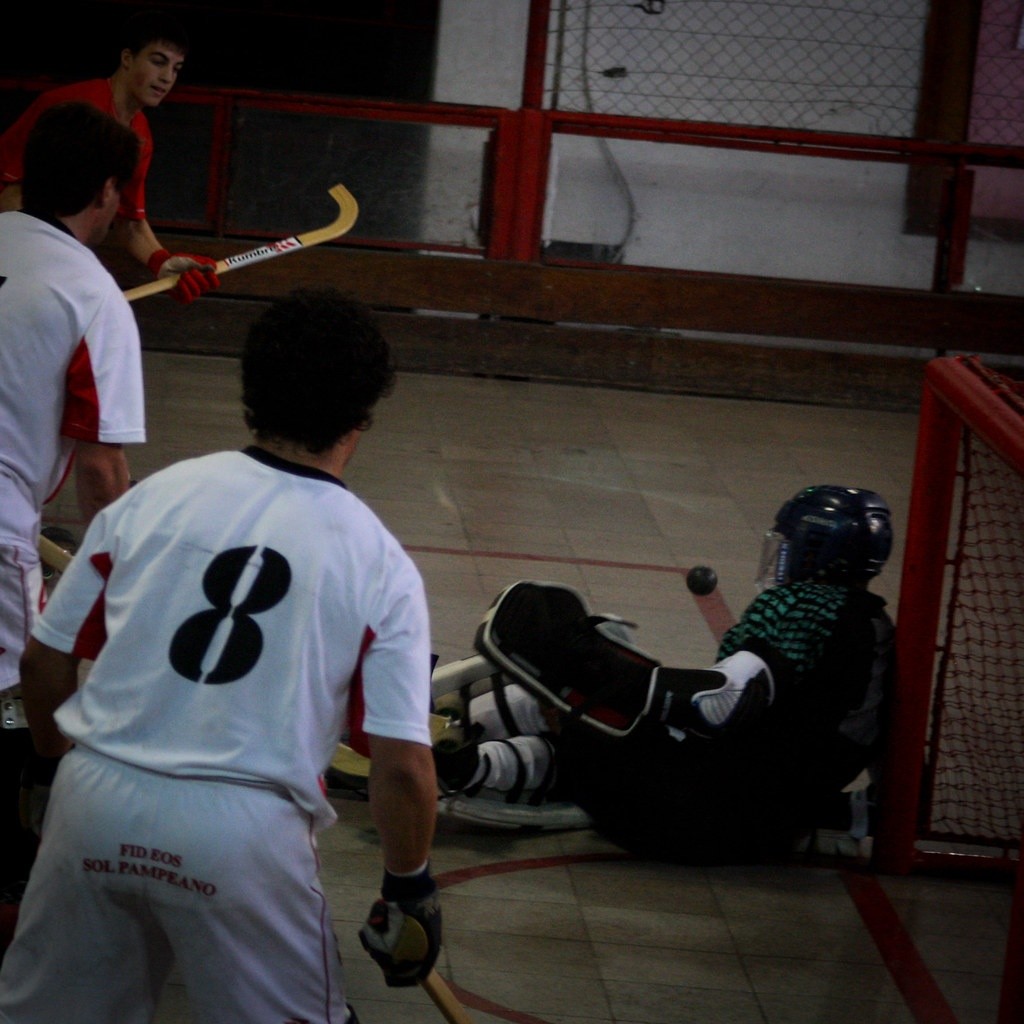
xmin=773 ymin=484 xmax=894 ymax=593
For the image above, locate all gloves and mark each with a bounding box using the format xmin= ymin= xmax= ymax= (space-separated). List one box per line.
xmin=146 ymin=249 xmax=220 ymax=306
xmin=359 ymin=864 xmax=441 ymax=988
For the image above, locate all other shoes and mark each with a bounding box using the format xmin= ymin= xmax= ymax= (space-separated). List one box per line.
xmin=321 ymin=696 xmax=482 ymax=807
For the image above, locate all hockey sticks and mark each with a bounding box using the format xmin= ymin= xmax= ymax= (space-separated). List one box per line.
xmin=367 ymin=897 xmax=474 ymax=1024
xmin=34 ymin=532 xmax=451 ymax=777
xmin=123 ymin=181 xmax=360 ymax=301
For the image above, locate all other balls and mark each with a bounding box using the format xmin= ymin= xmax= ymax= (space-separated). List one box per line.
xmin=685 ymin=564 xmax=718 ymax=596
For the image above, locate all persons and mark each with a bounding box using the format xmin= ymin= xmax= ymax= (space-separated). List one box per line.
xmin=0 ymin=14 xmax=224 ymax=307
xmin=435 ymin=481 xmax=897 ymax=862
xmin=0 ymin=286 xmax=444 ymax=1023
xmin=0 ymin=102 xmax=149 ymax=694
